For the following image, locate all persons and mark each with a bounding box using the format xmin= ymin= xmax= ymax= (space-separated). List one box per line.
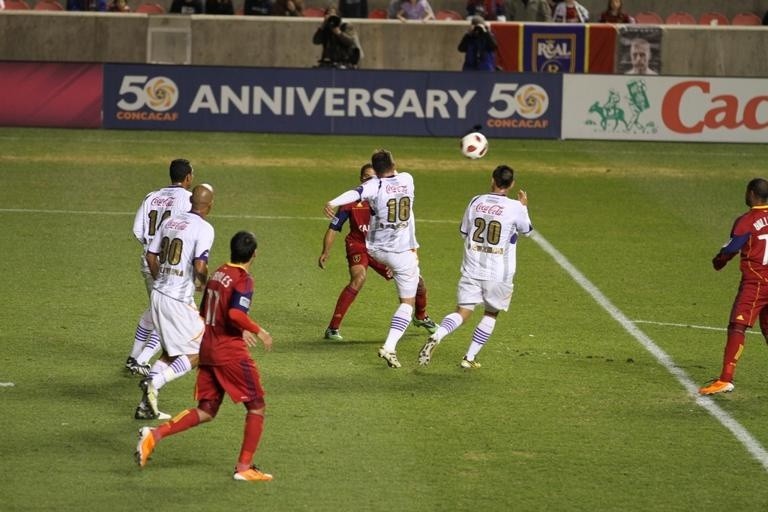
xmin=417 ymin=165 xmax=533 ymax=367
xmin=458 ymin=16 xmax=497 ymax=71
xmin=625 ymin=38 xmax=658 ymax=75
xmin=135 ymin=183 xmax=215 ymax=420
xmin=322 ymin=148 xmax=420 ymax=368
xmin=697 ymin=178 xmax=768 ymax=396
xmin=318 ymin=163 xmax=439 ymax=339
xmin=312 ymin=7 xmax=359 ymax=69
xmin=126 ymin=159 xmax=193 ymax=377
xmin=61 ymin=0 xmax=635 ymax=24
xmin=136 ymin=230 xmax=273 ymax=481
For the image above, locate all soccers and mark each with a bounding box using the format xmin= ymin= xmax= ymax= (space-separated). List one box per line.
xmin=461 ymin=133 xmax=488 ymax=159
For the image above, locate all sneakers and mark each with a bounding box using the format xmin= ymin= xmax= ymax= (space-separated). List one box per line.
xmin=325 ymin=328 xmax=343 ymax=340
xmin=413 ymin=315 xmax=439 ymax=333
xmin=418 ymin=336 xmax=438 ymax=366
xmin=698 ymin=380 xmax=734 ymax=395
xmin=233 ymin=466 xmax=273 ymax=481
xmin=378 ymin=344 xmax=402 ymax=368
xmin=134 ymin=426 xmax=156 ymax=467
xmin=125 ymin=355 xmax=172 ymax=420
xmin=460 ymin=356 xmax=481 ymax=368
